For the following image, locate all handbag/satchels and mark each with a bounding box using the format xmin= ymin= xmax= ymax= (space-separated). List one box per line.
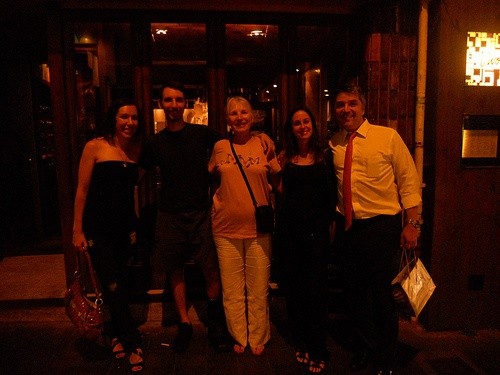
xmin=63 ymin=250 xmax=111 ymax=347
xmin=390 ymin=245 xmax=437 ymax=318
xmin=255 ymin=206 xmax=275 ymax=233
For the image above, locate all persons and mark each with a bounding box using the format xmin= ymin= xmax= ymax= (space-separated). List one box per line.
xmin=91 ymin=81 xmax=224 ymax=354
xmin=72 ymin=97 xmax=148 ymax=375
xmin=276 ymin=106 xmax=337 ymax=375
xmin=203 ymin=96 xmax=280 ymax=358
xmin=326 ymin=84 xmax=422 ymax=375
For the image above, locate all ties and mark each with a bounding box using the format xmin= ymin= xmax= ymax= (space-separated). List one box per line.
xmin=342 ymin=132 xmax=358 ymax=232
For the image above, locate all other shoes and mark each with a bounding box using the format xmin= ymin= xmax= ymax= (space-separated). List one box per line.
xmin=204 ymin=333 xmax=232 ymax=353
xmin=233 ymin=344 xmax=245 ymax=354
xmin=174 ymin=322 xmax=194 ymax=353
xmin=251 ymin=345 xmax=264 ymax=355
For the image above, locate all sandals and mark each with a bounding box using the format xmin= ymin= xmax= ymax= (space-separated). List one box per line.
xmin=307 ymin=358 xmax=326 ymax=375
xmin=295 ymin=350 xmax=309 ymax=366
xmin=110 ymin=338 xmax=128 ymax=361
xmin=127 ymin=344 xmax=145 ymax=374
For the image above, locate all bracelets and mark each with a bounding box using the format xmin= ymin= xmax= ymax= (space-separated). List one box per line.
xmin=408 ymin=217 xmax=421 ymax=230
